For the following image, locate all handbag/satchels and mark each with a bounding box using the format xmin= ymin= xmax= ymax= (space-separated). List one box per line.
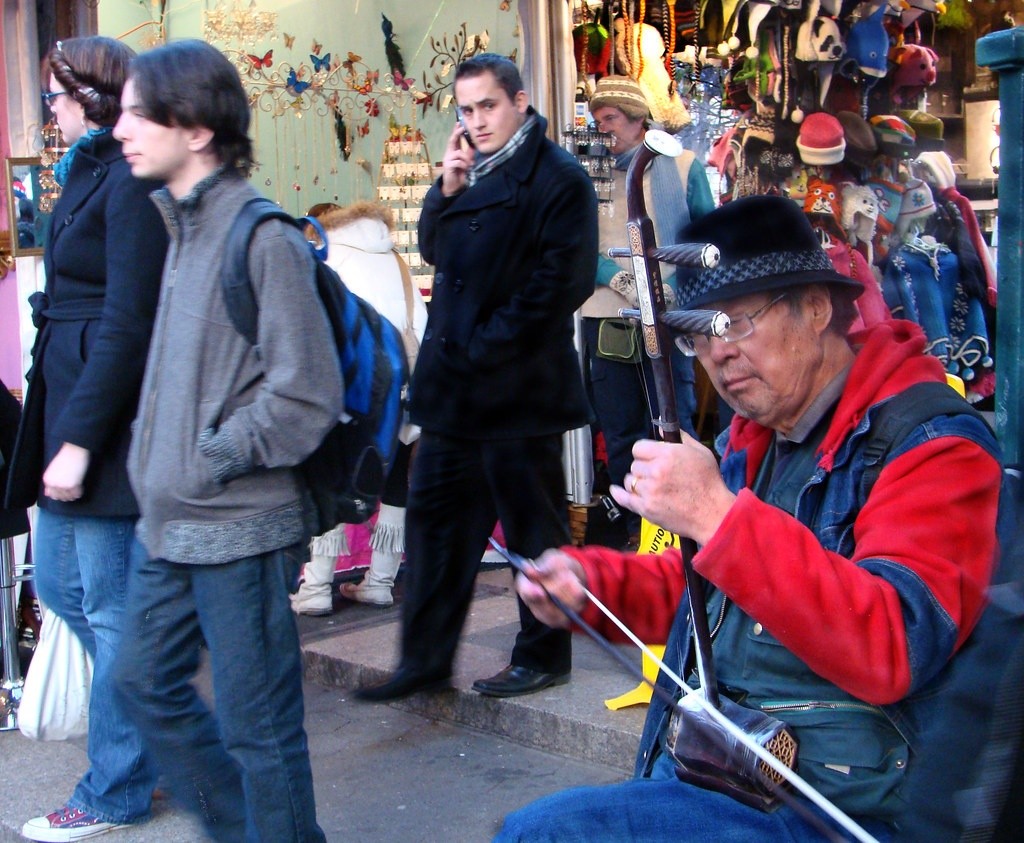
xmin=594 ymin=317 xmax=644 ymax=364
xmin=400 ymin=328 xmax=419 ymax=376
xmin=584 ymin=459 xmax=629 ymax=548
xmin=17 ymin=608 xmax=96 ymax=742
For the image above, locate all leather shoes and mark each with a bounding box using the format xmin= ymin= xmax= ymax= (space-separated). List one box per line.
xmin=350 ymin=663 xmax=451 ymax=704
xmin=471 ymin=663 xmax=570 ymax=697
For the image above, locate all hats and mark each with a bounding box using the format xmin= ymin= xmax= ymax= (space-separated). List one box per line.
xmin=677 ymin=195 xmax=865 ymax=302
xmin=569 ymin=0 xmax=956 ymax=281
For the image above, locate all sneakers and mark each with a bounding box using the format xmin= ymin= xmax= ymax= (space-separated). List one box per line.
xmin=22 ymin=803 xmax=136 ymax=842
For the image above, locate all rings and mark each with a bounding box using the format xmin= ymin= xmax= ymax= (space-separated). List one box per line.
xmin=631 ymin=477 xmax=640 ymax=493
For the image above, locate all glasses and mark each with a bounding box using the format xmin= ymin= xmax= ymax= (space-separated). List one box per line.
xmin=41 ymin=90 xmax=72 ymax=106
xmin=674 ymin=294 xmax=785 ymax=357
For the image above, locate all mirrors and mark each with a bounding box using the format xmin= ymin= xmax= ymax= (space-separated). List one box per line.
xmin=5 ymin=157 xmax=51 ymax=257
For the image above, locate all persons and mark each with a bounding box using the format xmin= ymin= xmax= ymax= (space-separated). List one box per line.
xmin=0 ymin=35 xmax=170 ymax=843
xmin=494 ymin=191 xmax=1024 ymax=843
xmin=351 ymin=52 xmax=601 ymax=701
xmin=113 ymin=39 xmax=326 ymax=843
xmin=291 ymin=203 xmax=427 ymax=616
xmin=583 ymin=77 xmax=715 ymax=550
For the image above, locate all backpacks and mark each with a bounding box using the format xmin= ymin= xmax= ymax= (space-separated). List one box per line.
xmin=218 ymin=199 xmax=410 ymax=535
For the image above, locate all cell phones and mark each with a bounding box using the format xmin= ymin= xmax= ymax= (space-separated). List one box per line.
xmin=456 ymin=106 xmax=476 ymax=150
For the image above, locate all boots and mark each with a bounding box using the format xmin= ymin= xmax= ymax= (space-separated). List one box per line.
xmin=289 ymin=521 xmax=351 ymax=615
xmin=339 ymin=501 xmax=407 ymax=605
